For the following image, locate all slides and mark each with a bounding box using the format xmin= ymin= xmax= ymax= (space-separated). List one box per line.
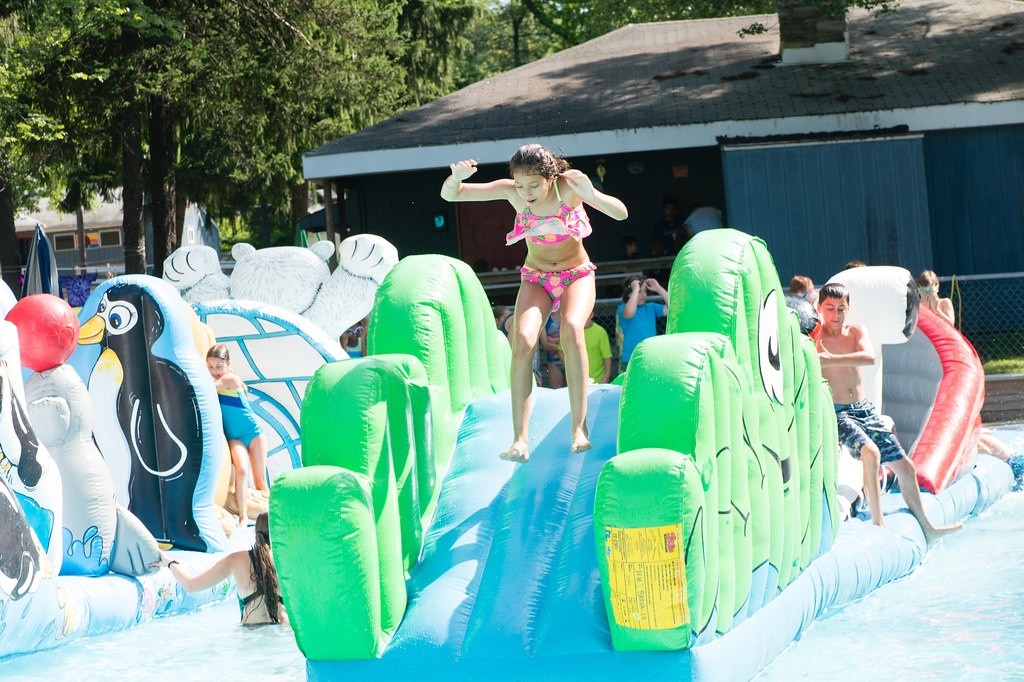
xmin=267 ymin=383 xmax=699 ymax=681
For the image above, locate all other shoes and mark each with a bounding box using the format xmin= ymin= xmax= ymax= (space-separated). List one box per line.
xmin=1011 ymin=455 xmax=1024 ymax=492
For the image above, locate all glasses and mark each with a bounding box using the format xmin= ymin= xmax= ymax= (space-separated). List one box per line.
xmin=919 ymin=288 xmax=935 ymax=295
xmin=347 ymin=326 xmax=364 ymax=337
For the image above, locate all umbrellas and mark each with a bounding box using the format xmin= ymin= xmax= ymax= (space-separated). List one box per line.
xmin=19 ymin=221 xmax=64 ymax=302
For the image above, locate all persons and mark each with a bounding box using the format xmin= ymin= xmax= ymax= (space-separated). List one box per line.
xmin=647 ymin=190 xmax=705 ymax=291
xmin=612 ymin=235 xmax=644 ymax=295
xmin=474 ymin=258 xmax=504 ymax=307
xmin=340 ymin=314 xmax=371 ymax=359
xmin=440 ymin=142 xmax=628 ymax=463
xmin=845 ymin=260 xmax=955 ymax=327
xmin=206 ymin=344 xmax=270 ymax=529
xmin=810 ymin=284 xmax=964 ymax=543
xmin=492 ymin=273 xmax=669 ymax=389
xmin=159 ymin=511 xmax=290 ymax=629
xmin=791 ymin=273 xmax=819 ymax=305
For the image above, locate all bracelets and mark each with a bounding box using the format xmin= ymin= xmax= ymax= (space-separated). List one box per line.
xmin=446 ymin=174 xmax=460 ymax=188
xmin=168 ymin=560 xmax=179 ymax=569
